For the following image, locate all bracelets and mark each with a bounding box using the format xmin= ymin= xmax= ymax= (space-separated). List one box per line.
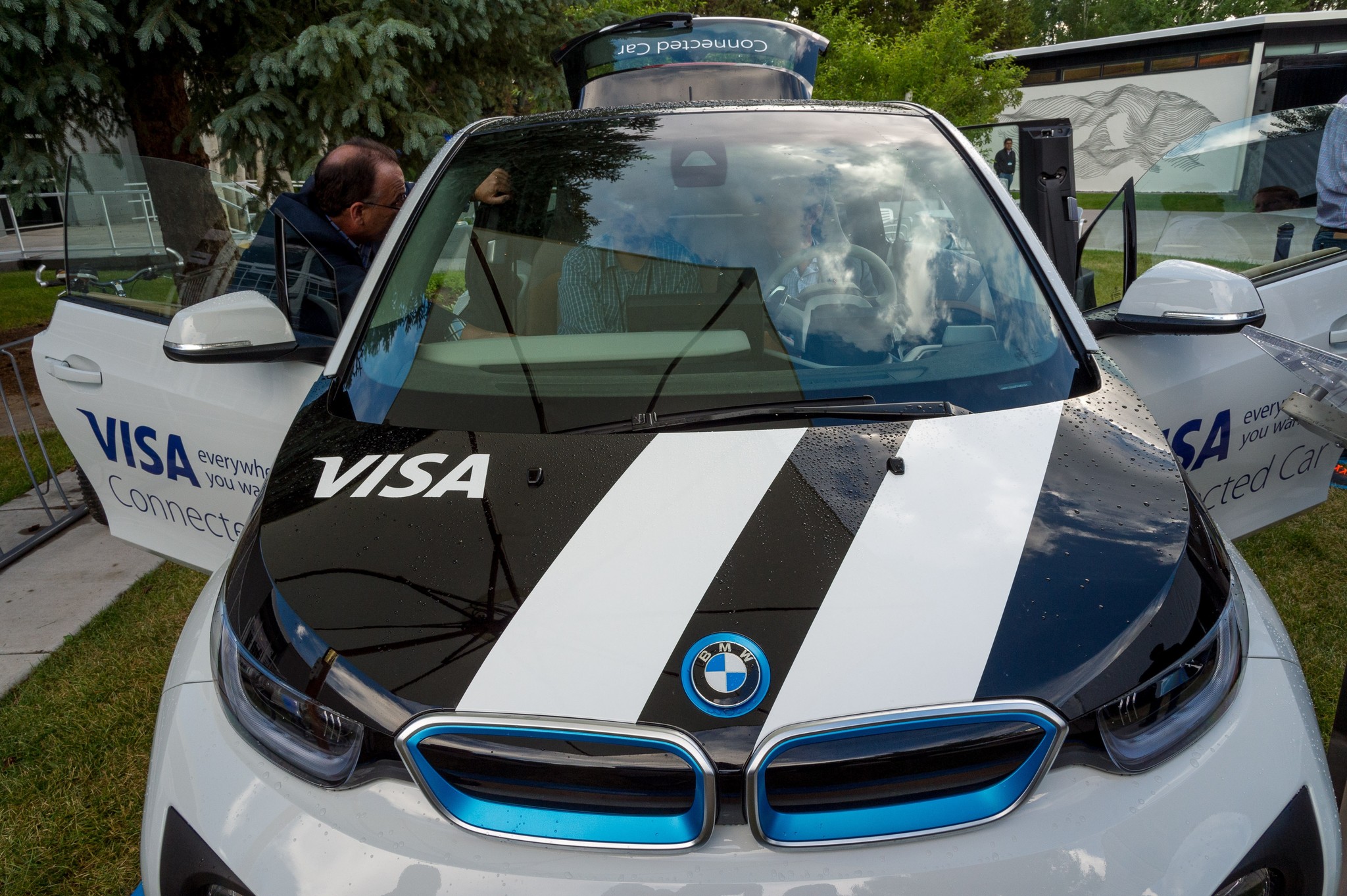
xmin=490 ymin=330 xmax=497 ymax=338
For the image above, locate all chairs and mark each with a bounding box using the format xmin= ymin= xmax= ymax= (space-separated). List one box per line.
xmin=730 ymin=177 xmax=861 ymax=287
xmin=525 ymin=188 xmax=611 ymax=334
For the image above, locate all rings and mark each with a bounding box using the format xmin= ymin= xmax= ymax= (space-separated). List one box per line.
xmin=495 ymin=175 xmax=499 ymax=179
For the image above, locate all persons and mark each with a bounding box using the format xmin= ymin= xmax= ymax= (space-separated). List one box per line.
xmin=559 ymin=157 xmax=705 ymax=335
xmin=729 ymin=158 xmax=893 ymax=324
xmin=994 ymin=138 xmax=1016 ymax=193
xmin=219 ymin=138 xmax=522 ymax=344
xmin=1312 ymin=95 xmax=1347 ymax=254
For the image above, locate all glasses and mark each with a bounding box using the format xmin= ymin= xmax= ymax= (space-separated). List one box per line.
xmin=346 ymin=195 xmax=407 ymax=209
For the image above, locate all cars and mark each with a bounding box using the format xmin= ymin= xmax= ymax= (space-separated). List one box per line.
xmin=25 ymin=8 xmax=1347 ymax=896
